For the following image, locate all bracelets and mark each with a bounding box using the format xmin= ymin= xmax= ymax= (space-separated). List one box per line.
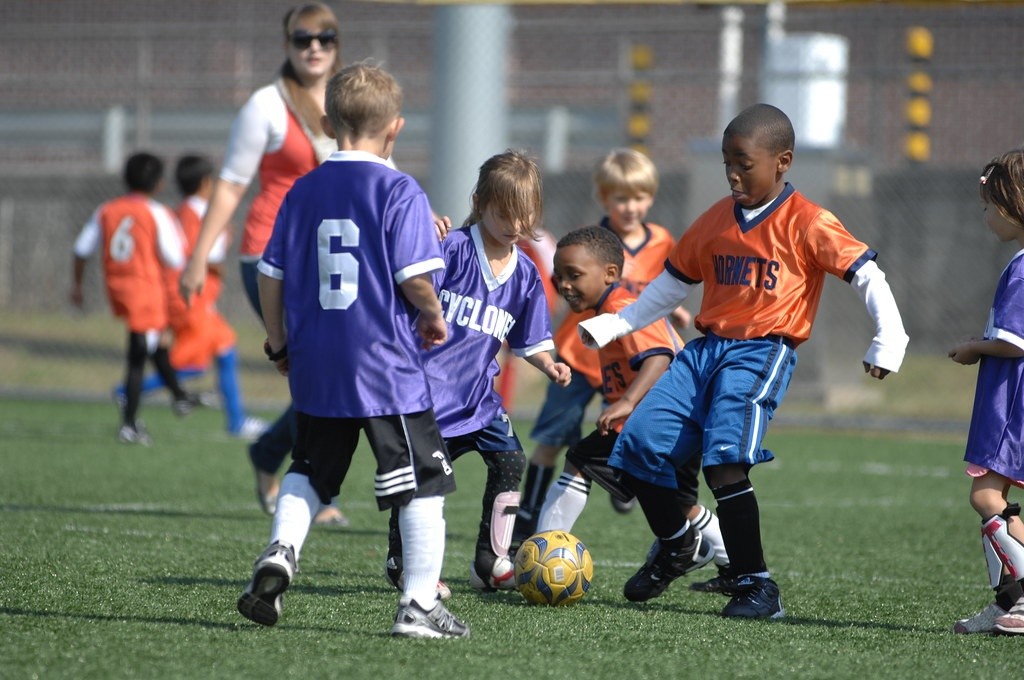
xmin=265 ymin=338 xmax=288 ymax=362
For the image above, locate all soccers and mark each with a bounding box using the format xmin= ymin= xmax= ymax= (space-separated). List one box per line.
xmin=514 ymin=529 xmax=594 ymax=607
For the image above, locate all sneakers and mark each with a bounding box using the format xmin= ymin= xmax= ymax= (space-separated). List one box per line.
xmin=991 ymin=596 xmax=1024 ymax=635
xmin=237 ymin=540 xmax=295 ymax=626
xmin=721 ymin=575 xmax=785 ymax=620
xmin=624 ymin=525 xmax=716 ymax=602
xmin=385 ymin=556 xmax=452 ymax=600
xmin=691 ymin=564 xmax=732 ymax=592
xmin=388 ymin=597 xmax=471 ymax=640
xmin=468 ymin=555 xmax=517 ymax=593
xmin=954 ymin=601 xmax=1009 ymax=635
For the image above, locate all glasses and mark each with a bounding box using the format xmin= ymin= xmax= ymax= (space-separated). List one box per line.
xmin=289 ymin=28 xmax=337 ymax=50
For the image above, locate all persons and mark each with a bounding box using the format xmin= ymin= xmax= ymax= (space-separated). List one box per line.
xmin=178 ymin=0 xmax=452 ymax=527
xmin=70 ymin=153 xmax=216 ymax=448
xmin=947 ymin=150 xmax=1024 ymax=634
xmin=382 ymin=153 xmax=572 ymax=592
xmin=508 ymin=150 xmax=692 ymax=542
xmin=499 ymin=227 xmax=560 ymax=413
xmin=538 ymin=228 xmax=732 ymax=591
xmin=577 ymin=103 xmax=910 ymax=618
xmin=109 ymin=156 xmax=273 ymax=440
xmin=235 ymin=61 xmax=468 ymax=640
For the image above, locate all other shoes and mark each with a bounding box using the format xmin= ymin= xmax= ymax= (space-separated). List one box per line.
xmin=314 ymin=514 xmax=348 ymax=527
xmin=237 ymin=417 xmax=268 ymax=439
xmin=255 ymin=466 xmax=278 ymax=515
xmin=119 ymin=427 xmax=149 ymax=445
